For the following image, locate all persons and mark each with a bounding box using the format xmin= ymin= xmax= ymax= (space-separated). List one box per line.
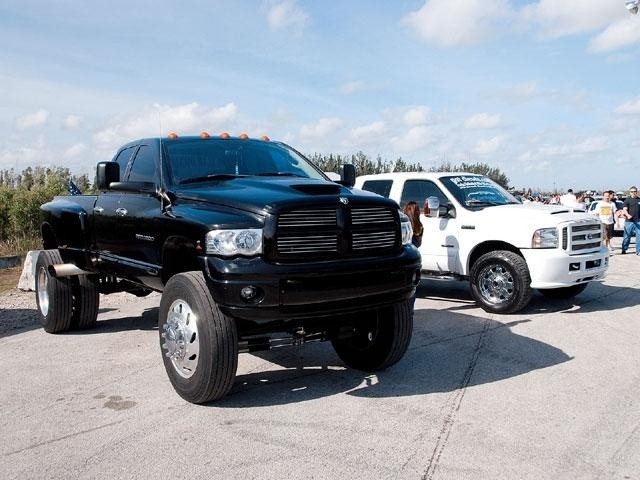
xmin=516 ymin=186 xmax=640 ymax=256
xmin=402 ymin=199 xmax=423 ymax=248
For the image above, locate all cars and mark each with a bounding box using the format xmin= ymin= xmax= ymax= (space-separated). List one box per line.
xmin=586 ymin=199 xmax=627 ymax=236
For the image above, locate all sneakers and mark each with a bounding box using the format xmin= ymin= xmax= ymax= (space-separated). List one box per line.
xmin=602 ymin=243 xmax=640 ymax=256
